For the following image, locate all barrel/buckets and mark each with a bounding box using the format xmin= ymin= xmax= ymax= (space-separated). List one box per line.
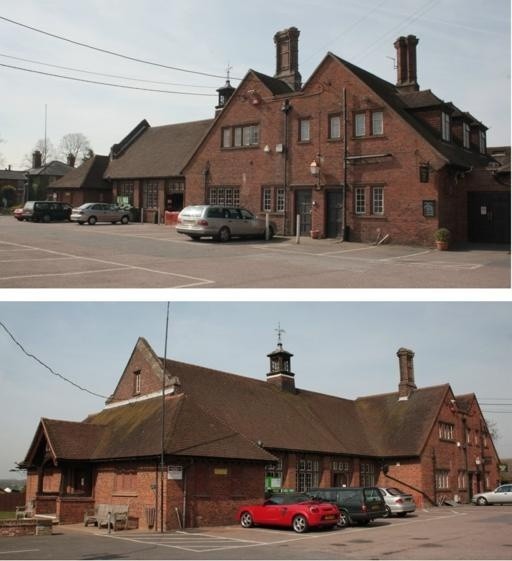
xmin=310 ymin=230 xmax=320 ymax=238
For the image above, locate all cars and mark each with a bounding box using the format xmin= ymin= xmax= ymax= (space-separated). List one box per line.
xmin=234 ymin=494 xmax=341 ymax=533
xmin=70 ymin=202 xmax=133 ymax=225
xmin=378 ymin=487 xmax=417 ymax=518
xmin=12 ymin=207 xmax=23 ymax=221
xmin=471 ymin=483 xmax=512 ymax=506
xmin=175 ymin=204 xmax=277 ymax=242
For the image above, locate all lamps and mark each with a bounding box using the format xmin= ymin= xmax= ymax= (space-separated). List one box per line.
xmin=310 ymin=161 xmax=319 ymax=191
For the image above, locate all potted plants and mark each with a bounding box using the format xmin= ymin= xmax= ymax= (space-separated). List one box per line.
xmin=434 ymin=227 xmax=451 ymax=250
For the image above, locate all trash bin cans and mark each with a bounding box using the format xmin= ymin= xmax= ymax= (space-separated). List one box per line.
xmin=310 ymin=230 xmax=320 ymax=239
xmin=153 ymin=212 xmax=158 ymax=223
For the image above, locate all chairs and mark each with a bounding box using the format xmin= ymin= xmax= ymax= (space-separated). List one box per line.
xmin=15 ymin=502 xmax=34 ymax=518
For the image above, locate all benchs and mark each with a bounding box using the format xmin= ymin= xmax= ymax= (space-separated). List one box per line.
xmin=84 ymin=503 xmax=129 ymax=531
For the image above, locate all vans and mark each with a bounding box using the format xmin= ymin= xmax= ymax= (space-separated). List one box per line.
xmin=23 ymin=201 xmax=73 ymax=222
xmin=303 ymin=486 xmax=387 ymax=526
